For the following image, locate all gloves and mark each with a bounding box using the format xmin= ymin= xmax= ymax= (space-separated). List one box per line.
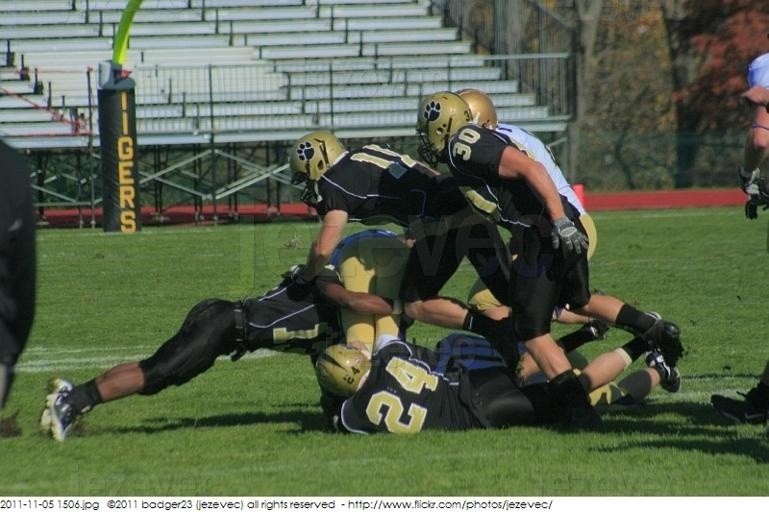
xmin=552 ymin=217 xmax=591 ymax=256
xmin=737 ymin=167 xmax=768 ymax=218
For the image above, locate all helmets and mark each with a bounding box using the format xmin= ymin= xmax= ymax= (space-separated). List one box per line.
xmin=317 ymin=345 xmax=372 ymax=395
xmin=417 ymin=90 xmax=497 ymax=164
xmin=288 ymin=132 xmax=350 ymax=208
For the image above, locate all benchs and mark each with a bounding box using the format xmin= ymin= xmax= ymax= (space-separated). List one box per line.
xmin=0 ymin=0 xmax=578 ymax=233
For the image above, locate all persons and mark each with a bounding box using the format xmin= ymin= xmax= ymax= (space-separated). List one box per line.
xmin=36 ymin=87 xmax=687 ymax=445
xmin=738 ymin=50 xmax=769 ymax=220
xmin=713 ymin=364 xmax=768 ymax=426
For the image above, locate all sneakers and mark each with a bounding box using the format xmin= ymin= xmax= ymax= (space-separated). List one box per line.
xmin=41 ymin=376 xmax=82 ymax=442
xmin=640 ymin=311 xmax=685 ymax=393
xmin=712 ymin=394 xmax=768 ymax=425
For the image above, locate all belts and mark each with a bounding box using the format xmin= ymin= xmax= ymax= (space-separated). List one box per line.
xmin=233 ymin=299 xmax=245 ymax=362
